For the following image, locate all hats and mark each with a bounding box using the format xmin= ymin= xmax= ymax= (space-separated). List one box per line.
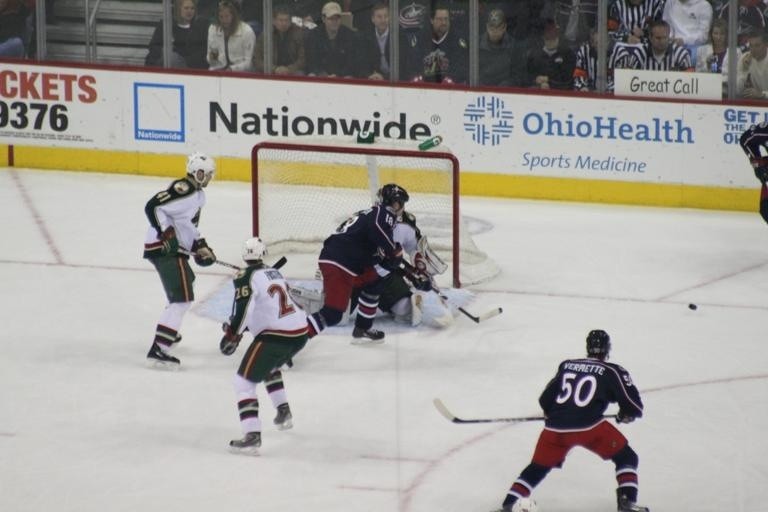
xmin=321 ymin=2 xmax=342 ymax=18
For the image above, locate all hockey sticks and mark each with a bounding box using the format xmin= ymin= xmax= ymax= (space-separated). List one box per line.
xmin=434 ymin=398 xmax=619 ymax=423
xmin=398 ymin=261 xmax=502 ymax=323
xmin=178 ymin=250 xmax=285 ymax=273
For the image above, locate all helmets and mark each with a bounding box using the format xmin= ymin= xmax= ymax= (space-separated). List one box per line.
xmin=186 ymin=154 xmax=214 ymax=175
xmin=377 ymin=184 xmax=409 ymax=207
xmin=242 ymin=237 xmax=266 ymax=261
xmin=586 ymin=329 xmax=610 ymax=358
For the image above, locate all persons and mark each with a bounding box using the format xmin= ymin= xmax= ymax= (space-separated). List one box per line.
xmin=373 ymin=187 xmax=421 ymax=322
xmin=740 ymin=122 xmax=768 ymax=225
xmin=0 ymin=1 xmax=31 ymax=59
xmin=307 ymin=183 xmax=432 ymax=338
xmin=220 ymin=236 xmax=311 ymax=449
xmin=145 ymin=0 xmax=767 ymax=99
xmin=502 ymin=330 xmax=647 ymax=512
xmin=142 ymin=152 xmax=217 ymax=363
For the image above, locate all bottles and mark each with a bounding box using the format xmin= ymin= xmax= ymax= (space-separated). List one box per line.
xmin=418 ymin=135 xmax=442 ymax=151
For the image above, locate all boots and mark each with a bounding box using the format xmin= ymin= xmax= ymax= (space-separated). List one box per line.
xmin=617 ymin=496 xmax=649 ymax=512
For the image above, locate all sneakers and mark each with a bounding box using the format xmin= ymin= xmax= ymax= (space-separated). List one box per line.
xmin=230 ymin=431 xmax=261 ymax=447
xmin=147 ymin=340 xmax=180 ymax=364
xmin=274 ymin=403 xmax=292 ymax=425
xmin=352 ymin=326 xmax=385 ymax=339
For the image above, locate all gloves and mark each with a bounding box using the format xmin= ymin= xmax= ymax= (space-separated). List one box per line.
xmin=220 ymin=326 xmax=243 ymax=355
xmin=191 ymin=238 xmax=215 ymax=266
xmin=157 ymin=225 xmax=179 ymax=257
xmin=752 ymin=158 xmax=766 ymax=184
xmin=406 ymin=268 xmax=433 ymax=290
xmin=386 ymin=242 xmax=404 ymax=264
xmin=616 ymin=411 xmax=634 ymax=423
